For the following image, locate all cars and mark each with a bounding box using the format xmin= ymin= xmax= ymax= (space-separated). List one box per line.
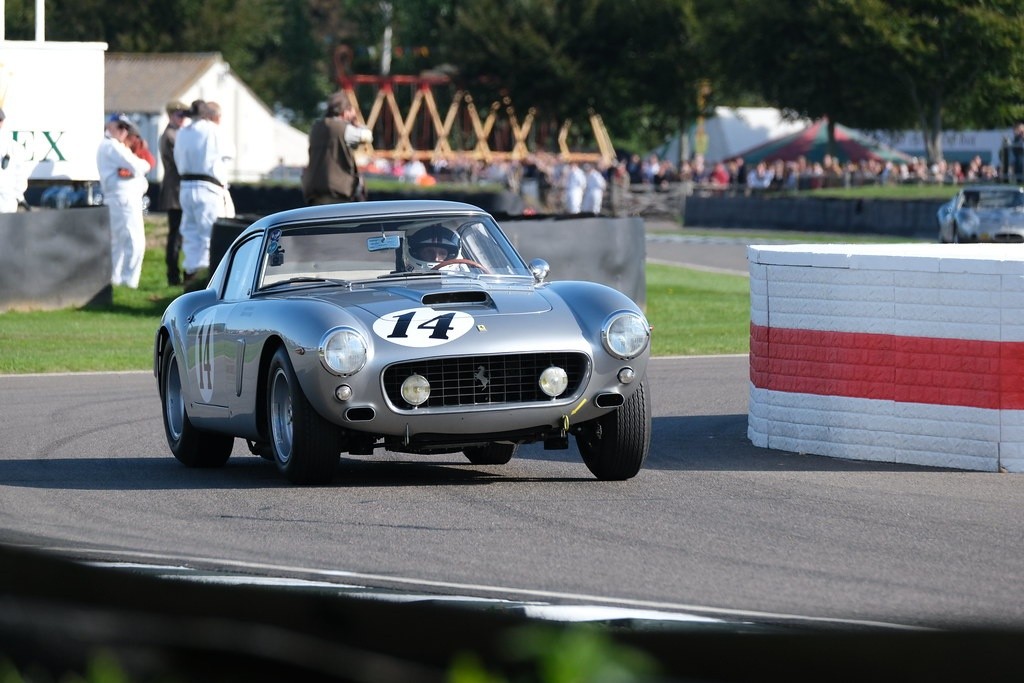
xmin=937 ymin=185 xmax=1024 ymax=244
xmin=150 ymin=199 xmax=654 ymax=482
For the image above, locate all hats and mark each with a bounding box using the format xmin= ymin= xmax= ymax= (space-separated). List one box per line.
xmin=166 ymin=101 xmax=190 ymax=113
xmin=110 ymin=111 xmax=132 ymax=127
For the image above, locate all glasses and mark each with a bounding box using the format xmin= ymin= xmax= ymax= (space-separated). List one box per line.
xmin=112 ymin=123 xmax=130 ymax=130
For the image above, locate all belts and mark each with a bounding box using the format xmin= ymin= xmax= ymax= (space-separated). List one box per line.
xmin=180 ymin=173 xmax=224 ymax=188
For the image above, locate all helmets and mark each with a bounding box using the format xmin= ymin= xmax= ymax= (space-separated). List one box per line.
xmin=402 ymin=220 xmax=462 ymax=272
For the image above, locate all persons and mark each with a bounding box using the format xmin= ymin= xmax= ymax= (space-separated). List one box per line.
xmin=158 ymin=100 xmax=236 ymax=286
xmin=371 ymin=154 xmax=607 ymax=214
xmin=300 ymin=93 xmax=374 ymax=206
xmin=1004 ymin=120 xmax=1024 ymax=185
xmin=615 ymin=153 xmax=999 ymax=189
xmin=402 ymin=226 xmax=463 ymax=271
xmin=96 ymin=112 xmax=156 ymax=289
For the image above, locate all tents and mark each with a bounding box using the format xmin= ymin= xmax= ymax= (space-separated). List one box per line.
xmin=723 ymin=116 xmax=914 ymax=172
